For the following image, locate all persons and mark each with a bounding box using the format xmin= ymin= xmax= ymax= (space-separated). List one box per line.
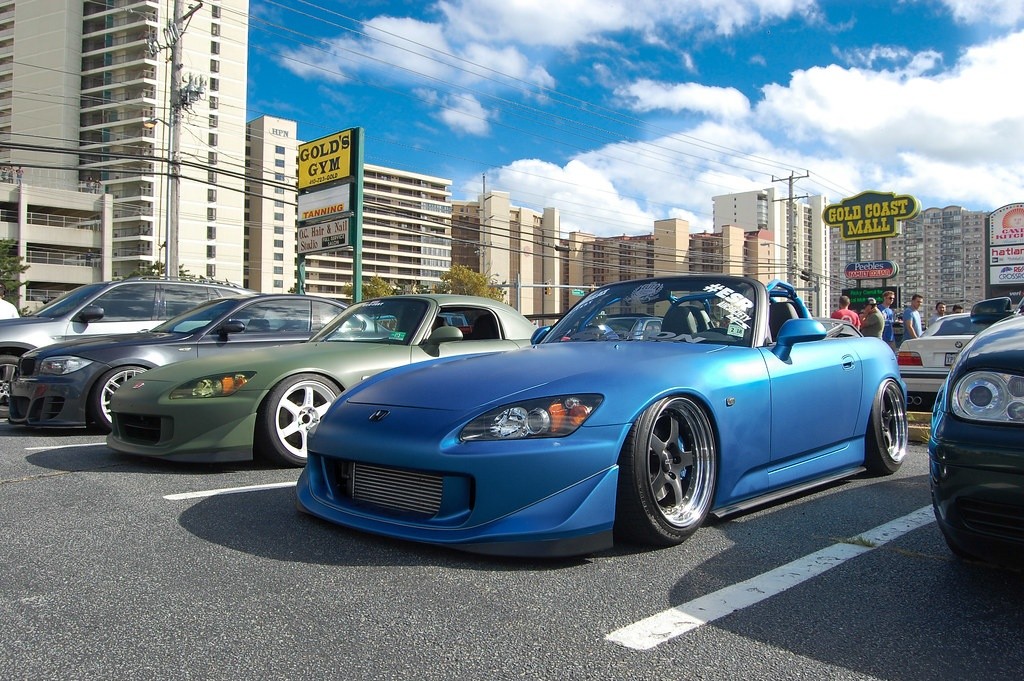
xmin=85 ymin=249 xmax=93 ymax=266
xmin=952 ymin=304 xmax=963 ymax=314
xmin=903 ymin=294 xmax=923 ymax=342
xmin=830 ymin=297 xmax=884 ymax=341
xmin=0 ymin=283 xmax=21 ymax=406
xmin=87 ymin=176 xmax=102 ymax=194
xmin=929 ymin=302 xmax=946 ymax=326
xmin=2 ymin=165 xmax=24 ymax=184
xmin=877 ymin=291 xmax=904 ymax=353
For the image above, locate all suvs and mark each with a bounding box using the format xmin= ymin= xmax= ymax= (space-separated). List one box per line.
xmin=0 ymin=276 xmax=265 ymax=422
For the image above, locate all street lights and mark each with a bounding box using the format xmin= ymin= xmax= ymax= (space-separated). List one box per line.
xmin=760 ymin=242 xmax=795 ymax=286
xmin=144 ymin=118 xmax=180 ymax=282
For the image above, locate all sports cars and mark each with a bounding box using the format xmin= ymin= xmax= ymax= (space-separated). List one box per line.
xmin=106 ymin=293 xmax=568 ymax=472
xmin=296 ymin=273 xmax=910 ymax=557
xmin=7 ymin=293 xmax=392 ymax=431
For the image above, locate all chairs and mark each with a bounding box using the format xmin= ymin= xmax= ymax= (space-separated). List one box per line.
xmin=768 ymin=301 xmax=799 ymax=342
xmin=471 ymin=315 xmax=499 ymax=338
xmin=245 ymin=319 xmax=270 ymax=333
xmin=662 ymin=305 xmax=711 ymax=337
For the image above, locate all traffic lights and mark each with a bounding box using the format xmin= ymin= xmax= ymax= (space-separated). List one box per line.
xmin=544 ymin=282 xmax=549 ymax=296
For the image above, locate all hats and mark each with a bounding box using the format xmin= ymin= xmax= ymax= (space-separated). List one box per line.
xmin=861 ymin=298 xmax=877 ymax=309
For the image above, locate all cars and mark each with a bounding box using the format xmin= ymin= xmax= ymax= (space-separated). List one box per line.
xmin=899 ymin=313 xmax=989 ymax=411
xmin=927 ymin=296 xmax=1023 ymax=578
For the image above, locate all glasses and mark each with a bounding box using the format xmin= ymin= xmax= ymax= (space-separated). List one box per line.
xmin=885 ymin=297 xmax=895 ymax=300
